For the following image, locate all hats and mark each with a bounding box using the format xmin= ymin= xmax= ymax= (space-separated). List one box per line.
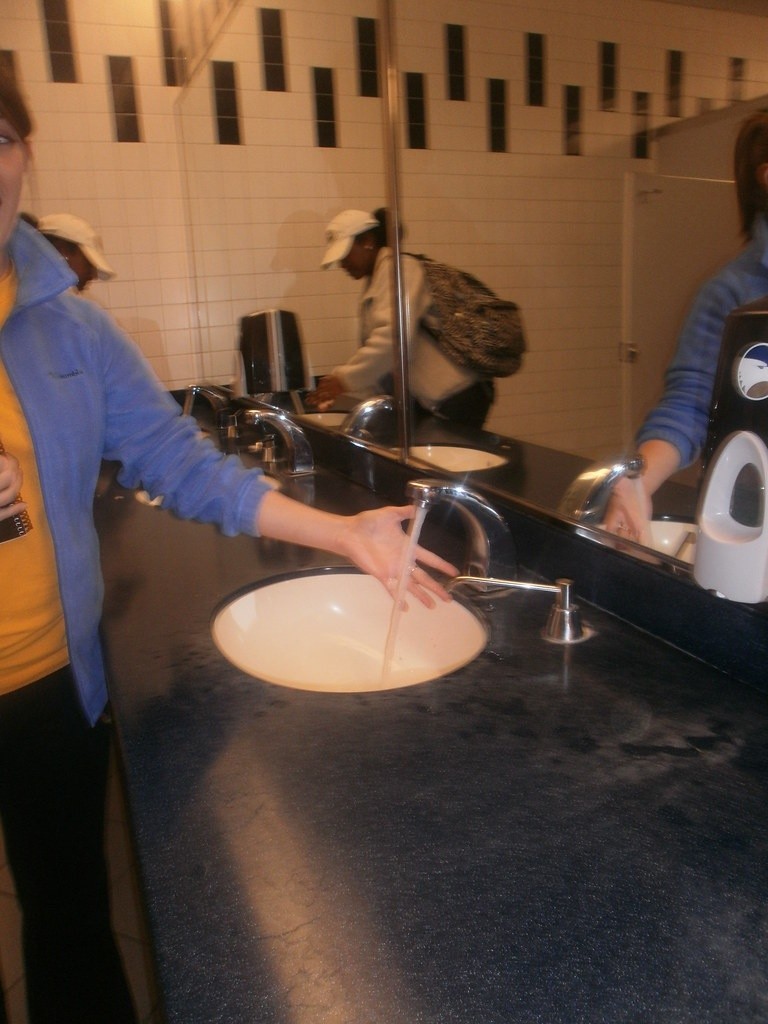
xmin=321 ymin=210 xmax=381 ymax=270
xmin=38 ymin=214 xmax=116 ymax=282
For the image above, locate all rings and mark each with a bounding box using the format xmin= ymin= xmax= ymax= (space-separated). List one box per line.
xmin=619 ymin=524 xmax=629 ymax=531
xmin=410 ymin=565 xmax=418 ymax=574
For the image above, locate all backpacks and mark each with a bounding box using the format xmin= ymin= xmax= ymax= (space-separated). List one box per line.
xmin=392 ymin=252 xmax=525 ymax=378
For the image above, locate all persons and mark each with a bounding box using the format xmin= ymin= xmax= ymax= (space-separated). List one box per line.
xmin=0 ymin=64 xmax=464 ymax=1023
xmin=602 ymin=105 xmax=768 ymax=550
xmin=303 ymin=205 xmax=525 ymax=433
xmin=17 ymin=209 xmax=116 ymax=296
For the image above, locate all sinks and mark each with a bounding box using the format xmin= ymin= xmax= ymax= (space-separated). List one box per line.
xmin=133 ymin=470 xmax=282 ymax=509
xmin=300 ymin=412 xmax=352 ymax=428
xmin=595 ymin=515 xmax=699 ymax=566
xmin=207 ymin=565 xmax=492 ymax=695
xmin=390 ymin=442 xmax=511 ymax=473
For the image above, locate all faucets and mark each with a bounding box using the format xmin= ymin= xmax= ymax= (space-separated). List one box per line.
xmin=558 ymin=451 xmax=648 ymax=531
xmin=339 ymin=394 xmax=397 ymax=443
xmin=185 ymin=383 xmax=230 ymax=423
xmin=243 ymin=409 xmax=315 ymax=474
xmin=408 ymin=478 xmax=526 ymax=594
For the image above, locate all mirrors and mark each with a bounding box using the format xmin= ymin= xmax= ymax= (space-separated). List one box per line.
xmin=176 ymin=0 xmax=768 ymax=563
